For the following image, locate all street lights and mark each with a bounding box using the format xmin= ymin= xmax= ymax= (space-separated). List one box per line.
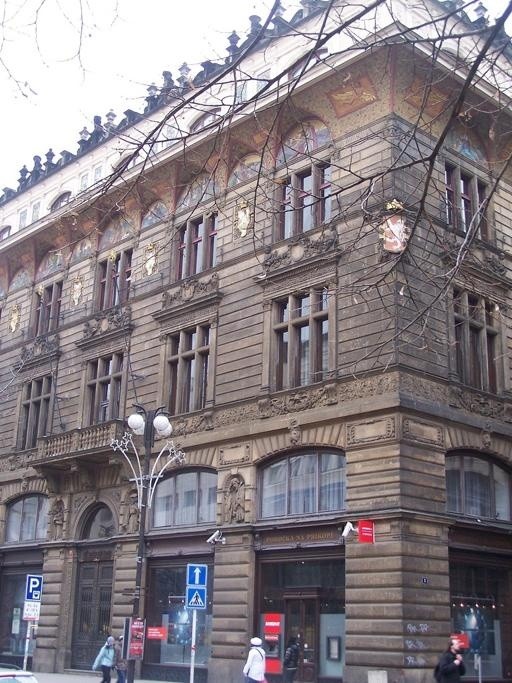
xmin=100 ymin=395 xmax=188 ymax=680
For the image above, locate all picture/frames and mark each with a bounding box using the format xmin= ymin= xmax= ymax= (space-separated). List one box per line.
xmin=325 ymin=633 xmax=342 ymax=663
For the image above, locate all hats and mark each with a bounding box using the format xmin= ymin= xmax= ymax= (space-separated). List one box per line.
xmin=107 ymin=636 xmax=114 ymax=644
xmin=250 ymin=636 xmax=263 ymax=646
xmin=119 ymin=635 xmax=125 ymax=641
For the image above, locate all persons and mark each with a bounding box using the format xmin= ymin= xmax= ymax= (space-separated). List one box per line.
xmin=92 ymin=636 xmax=116 ymax=683
xmin=243 ymin=636 xmax=266 ymax=683
xmin=434 ymin=638 xmax=466 ymax=683
xmin=113 ymin=635 xmax=128 ymax=683
xmin=281 ymin=636 xmax=299 ymax=683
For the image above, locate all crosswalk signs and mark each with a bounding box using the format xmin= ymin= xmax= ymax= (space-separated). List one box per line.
xmin=183 ymin=585 xmax=207 ymax=607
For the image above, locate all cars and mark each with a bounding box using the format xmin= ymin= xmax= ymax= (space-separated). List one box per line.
xmin=0 ymin=663 xmax=42 ymax=682
